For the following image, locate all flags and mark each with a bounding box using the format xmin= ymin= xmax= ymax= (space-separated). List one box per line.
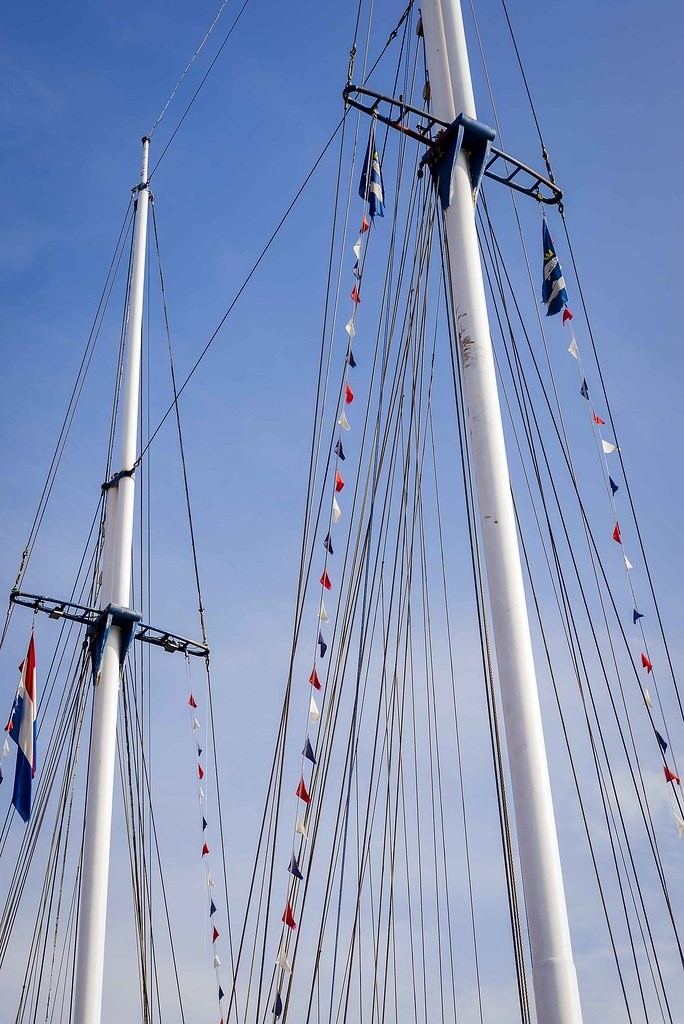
xmin=541 ymin=219 xmax=569 ymax=316
xmin=5 ymin=632 xmax=37 ymax=819
xmin=358 ymin=125 xmax=386 ymax=230
